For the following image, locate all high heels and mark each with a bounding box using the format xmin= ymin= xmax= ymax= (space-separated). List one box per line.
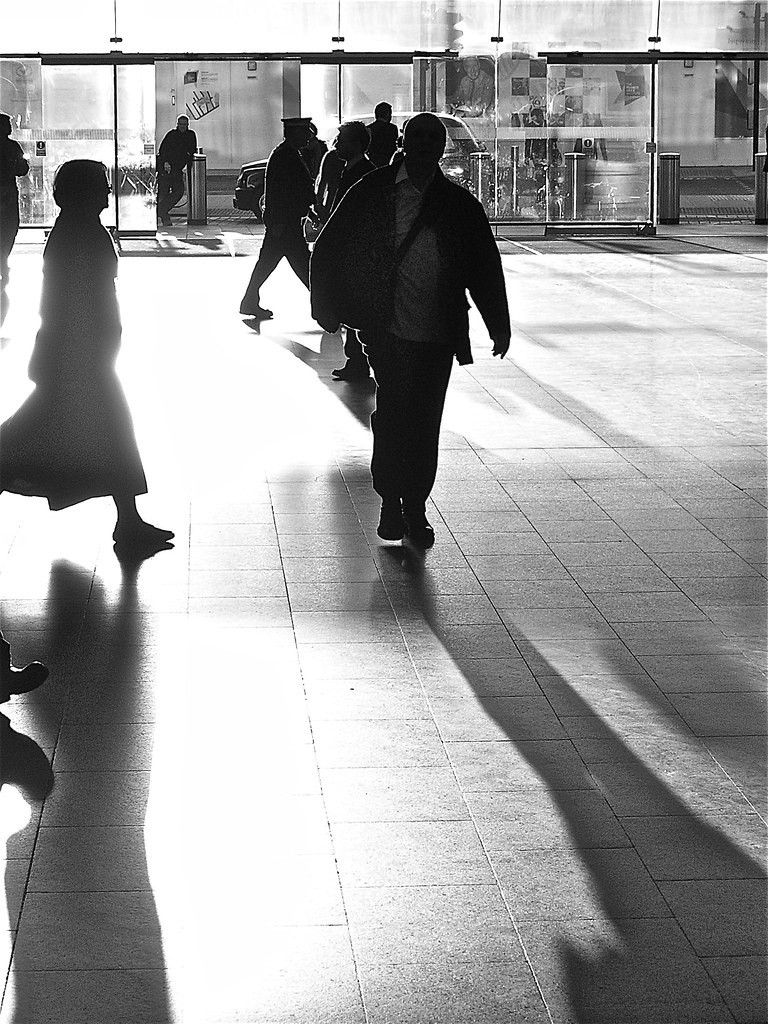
xmin=0 ymin=656 xmax=48 ymax=706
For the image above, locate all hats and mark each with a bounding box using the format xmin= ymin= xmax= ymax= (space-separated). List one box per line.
xmin=280 ymin=115 xmax=313 ymax=128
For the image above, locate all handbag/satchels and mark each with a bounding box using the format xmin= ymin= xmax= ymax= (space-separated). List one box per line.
xmin=331 ymin=243 xmax=395 ymax=330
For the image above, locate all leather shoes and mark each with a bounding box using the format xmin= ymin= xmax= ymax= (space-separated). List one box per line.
xmin=239 ymin=300 xmax=273 ymax=322
xmin=330 ymin=355 xmax=371 ymax=381
xmin=112 ymin=525 xmax=174 ymax=544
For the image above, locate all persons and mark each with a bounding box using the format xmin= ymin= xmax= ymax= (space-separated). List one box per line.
xmin=238 ymin=117 xmax=316 ymax=318
xmin=325 ymin=121 xmax=378 ymax=379
xmin=315 ymin=136 xmax=346 ymax=222
xmin=0 ymin=159 xmax=175 ymax=560
xmin=573 ymin=113 xmax=608 ymax=205
xmin=156 ymin=114 xmax=197 ymax=227
xmin=364 ymin=102 xmax=399 ymax=167
xmin=447 ymin=57 xmax=495 ymax=119
xmin=524 ymin=109 xmax=559 ymax=208
xmin=0 ymin=114 xmax=30 ymax=277
xmin=299 ymin=120 xmax=328 ymax=230
xmin=309 ymin=112 xmax=512 ymax=550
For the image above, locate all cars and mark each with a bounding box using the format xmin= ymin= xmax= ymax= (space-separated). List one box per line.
xmin=230 ymin=111 xmax=496 ymax=222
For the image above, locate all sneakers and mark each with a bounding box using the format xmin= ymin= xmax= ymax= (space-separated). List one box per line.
xmin=399 ymin=503 xmax=435 ymax=540
xmin=374 ymin=494 xmax=406 ymax=541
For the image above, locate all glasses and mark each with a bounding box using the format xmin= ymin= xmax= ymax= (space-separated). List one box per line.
xmin=177 ymin=123 xmax=188 ymax=126
